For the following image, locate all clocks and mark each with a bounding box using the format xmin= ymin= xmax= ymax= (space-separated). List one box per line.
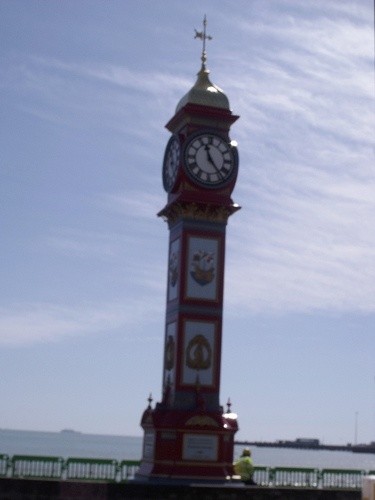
xmin=161 ymin=134 xmax=182 ymax=193
xmin=182 ymin=130 xmax=238 ymax=191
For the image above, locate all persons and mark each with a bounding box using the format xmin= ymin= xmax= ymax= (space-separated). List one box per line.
xmin=234 ymin=448 xmax=260 ymax=486
